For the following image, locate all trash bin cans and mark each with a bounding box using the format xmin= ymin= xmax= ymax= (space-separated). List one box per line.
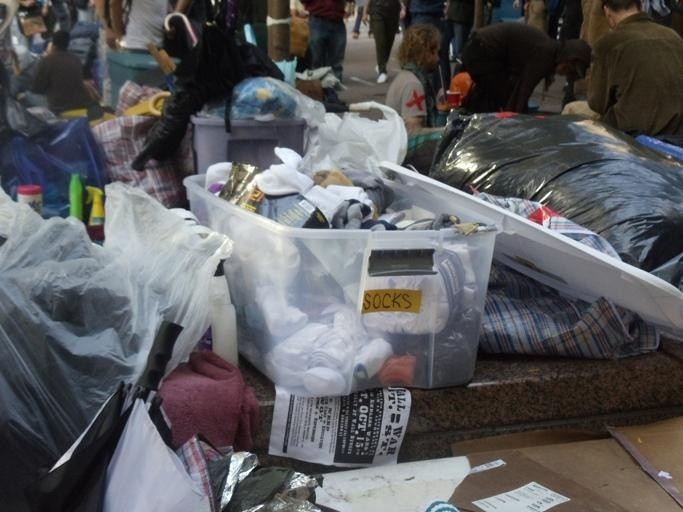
xmin=105 ymin=53 xmax=180 ymax=110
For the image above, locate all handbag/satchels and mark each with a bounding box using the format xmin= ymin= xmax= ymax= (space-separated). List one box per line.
xmin=91 ymin=80 xmax=196 ymax=208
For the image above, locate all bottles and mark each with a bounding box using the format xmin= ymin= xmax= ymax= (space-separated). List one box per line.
xmin=15 ymin=185 xmax=42 ymax=215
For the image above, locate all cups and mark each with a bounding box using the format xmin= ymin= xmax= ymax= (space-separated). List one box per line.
xmin=448 ymin=91 xmax=460 ymax=107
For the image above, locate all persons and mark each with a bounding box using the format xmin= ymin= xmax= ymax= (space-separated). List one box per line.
xmin=0 ymin=1 xmax=682 ymax=138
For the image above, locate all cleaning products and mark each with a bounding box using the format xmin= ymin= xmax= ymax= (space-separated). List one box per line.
xmin=200 ymin=259 xmax=240 ymax=366
xmin=85 ymin=186 xmax=106 ymax=241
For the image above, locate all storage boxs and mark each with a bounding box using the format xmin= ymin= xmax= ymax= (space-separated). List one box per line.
xmin=184 ymin=168 xmax=505 ymax=402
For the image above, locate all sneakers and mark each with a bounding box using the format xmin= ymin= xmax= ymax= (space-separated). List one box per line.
xmin=377 ymin=73 xmax=389 ymax=84
